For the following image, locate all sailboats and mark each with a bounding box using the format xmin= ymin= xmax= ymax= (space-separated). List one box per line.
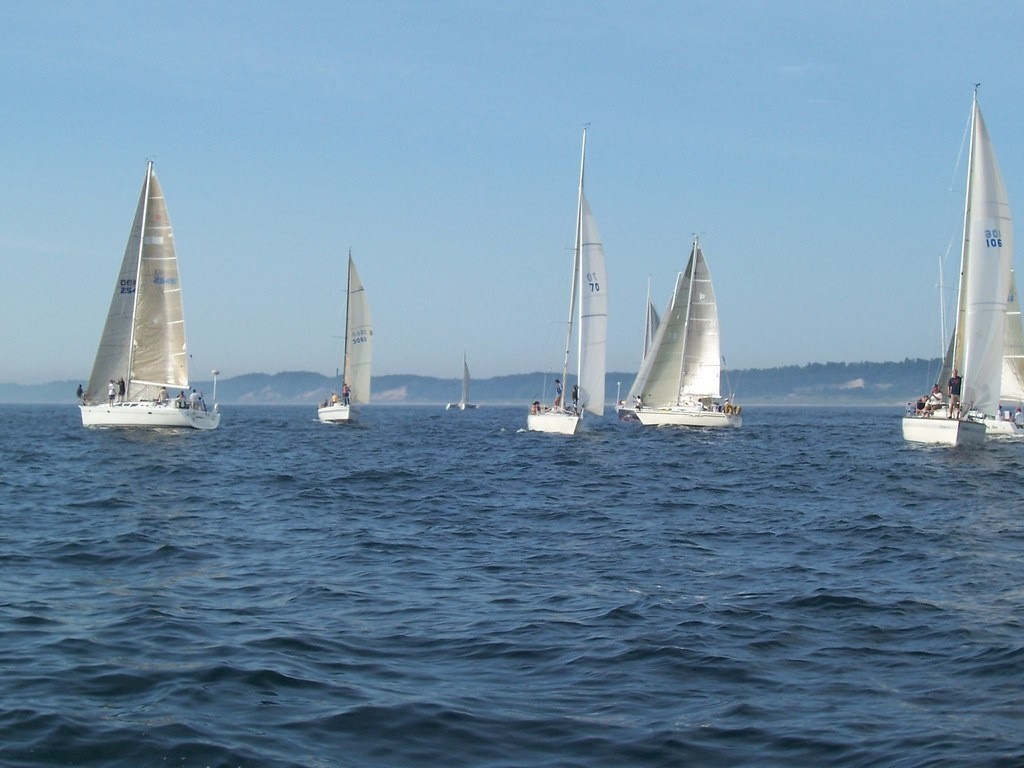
xmin=527 ymin=122 xmax=608 ymax=437
xmin=614 ymin=231 xmax=743 ymax=429
xmin=900 ymin=82 xmax=1024 ymax=447
xmin=444 ymin=347 xmax=482 ymax=412
xmin=316 ymin=246 xmax=374 ymax=425
xmin=79 ymin=161 xmax=222 ymax=429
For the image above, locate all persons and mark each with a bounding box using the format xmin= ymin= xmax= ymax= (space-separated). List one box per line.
xmin=1014 ymin=407 xmax=1024 ymax=429
xmin=177 ymin=391 xmax=186 ymax=408
xmin=77 ymin=384 xmax=83 ymax=398
xmin=331 ymin=393 xmax=337 ymax=403
xmin=190 ymin=389 xmax=199 ymax=403
xmin=948 ymin=369 xmax=962 ymax=419
xmin=109 ymin=380 xmax=117 ymax=407
xmin=118 ymin=378 xmax=125 ymax=402
xmin=916 ymin=384 xmax=942 ymax=418
xmin=159 ymin=387 xmax=169 ymax=403
xmin=554 ymin=379 xmax=562 ymax=406
xmin=572 ymin=385 xmax=577 ymax=410
xmin=531 ymin=401 xmax=540 ymax=414
xmin=696 ymin=399 xmax=703 ymax=408
xmin=343 ymin=384 xmax=351 ymax=406
xmin=197 ymin=397 xmax=203 ymax=405
xmin=724 ymin=398 xmax=728 ymax=405
xmin=633 ymin=395 xmax=642 ymax=407
